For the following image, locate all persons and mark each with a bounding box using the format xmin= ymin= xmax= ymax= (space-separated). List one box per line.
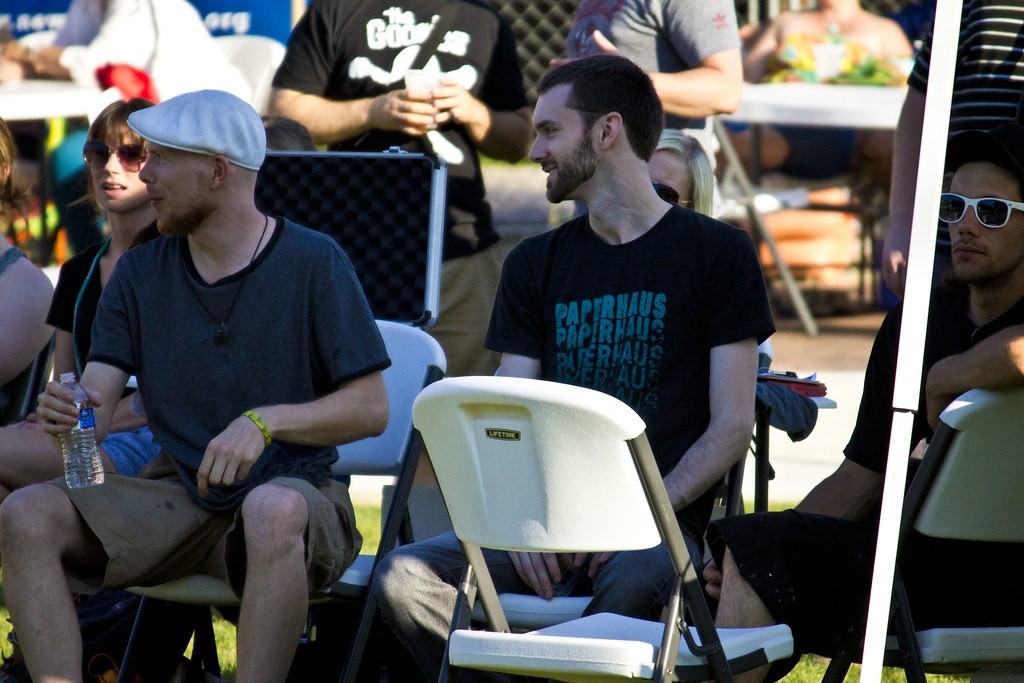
xmin=0 ymin=1 xmax=744 ymax=262
xmin=0 ymin=90 xmax=393 ymax=683
xmin=703 ymin=0 xmax=1024 ymax=683
xmin=647 ymin=129 xmax=713 ymax=221
xmin=371 ymin=50 xmax=779 ymax=683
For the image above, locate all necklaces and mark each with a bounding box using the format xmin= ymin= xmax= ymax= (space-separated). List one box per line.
xmin=181 ymin=213 xmax=268 ymax=346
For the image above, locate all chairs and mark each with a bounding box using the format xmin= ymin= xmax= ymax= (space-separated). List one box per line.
xmin=412 ymin=373 xmax=795 ymax=683
xmin=459 ymin=336 xmax=777 ymax=628
xmin=816 ymin=383 xmax=1024 ymax=683
xmin=109 ymin=318 xmax=448 ymax=681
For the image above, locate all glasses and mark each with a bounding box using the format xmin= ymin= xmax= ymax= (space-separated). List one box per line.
xmin=939 ymin=191 xmax=1024 ymax=228
xmin=652 ymin=183 xmax=693 ymax=207
xmin=81 ymin=140 xmax=149 ymax=173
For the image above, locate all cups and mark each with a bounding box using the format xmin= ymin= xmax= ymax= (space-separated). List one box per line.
xmin=405 ymin=69 xmax=443 ymax=130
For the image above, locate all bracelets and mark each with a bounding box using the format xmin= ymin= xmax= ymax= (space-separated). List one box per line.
xmin=241 ymin=411 xmax=272 ymax=447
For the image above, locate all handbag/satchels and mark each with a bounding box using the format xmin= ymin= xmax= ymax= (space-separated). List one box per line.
xmin=1 ymin=590 xmax=201 ymax=682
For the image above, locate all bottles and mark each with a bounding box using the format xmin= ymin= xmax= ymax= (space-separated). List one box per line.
xmin=57 ymin=372 xmax=105 ymax=489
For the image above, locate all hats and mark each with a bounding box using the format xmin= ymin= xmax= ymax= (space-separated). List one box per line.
xmin=945 ymin=123 xmax=1024 ymax=188
xmin=126 ymin=89 xmax=266 ymax=170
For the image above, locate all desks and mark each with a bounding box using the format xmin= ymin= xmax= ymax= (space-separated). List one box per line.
xmin=696 ymin=82 xmax=906 ymax=332
xmin=0 ymin=75 xmax=105 ymax=263
